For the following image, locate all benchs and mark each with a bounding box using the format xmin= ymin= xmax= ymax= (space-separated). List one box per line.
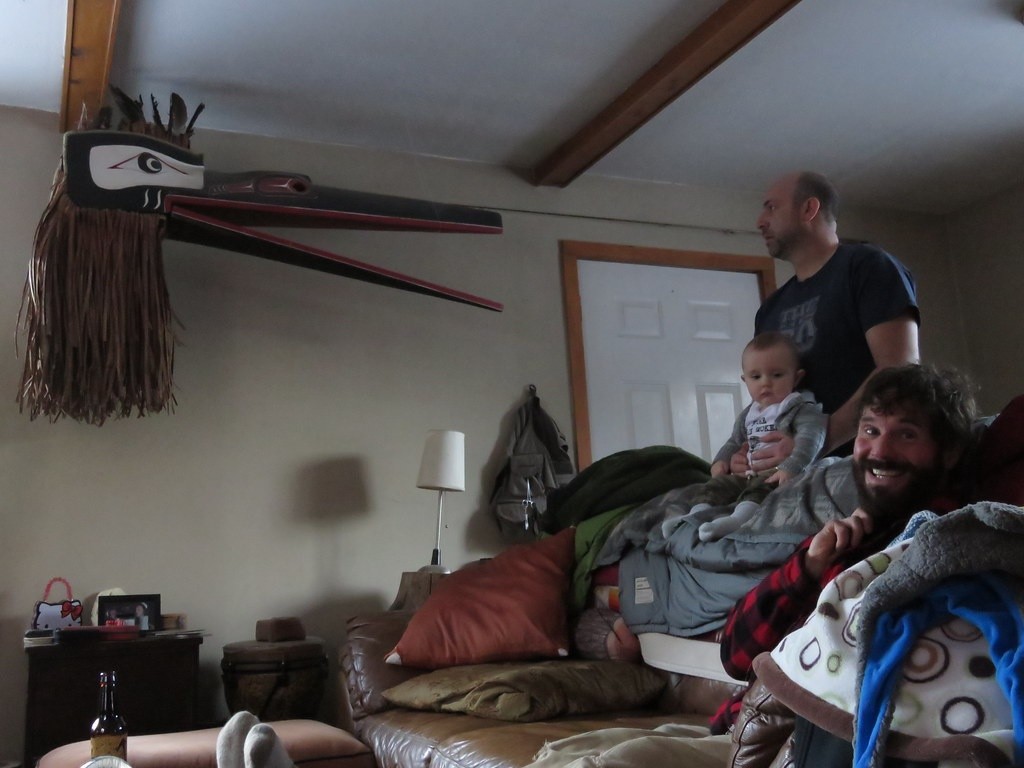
xmin=35 ymin=718 xmax=373 ymax=768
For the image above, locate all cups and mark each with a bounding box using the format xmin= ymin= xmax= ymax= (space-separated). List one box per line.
xmin=160 ymin=613 xmax=188 ymax=630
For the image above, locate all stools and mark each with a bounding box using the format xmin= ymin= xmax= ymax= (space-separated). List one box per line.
xmin=219 ymin=637 xmax=330 ymax=726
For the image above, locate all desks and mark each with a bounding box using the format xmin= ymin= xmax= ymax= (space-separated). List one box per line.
xmin=388 ymin=572 xmax=455 ymax=612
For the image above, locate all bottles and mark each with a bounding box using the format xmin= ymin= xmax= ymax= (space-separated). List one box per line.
xmin=90 ymin=670 xmax=128 ymax=763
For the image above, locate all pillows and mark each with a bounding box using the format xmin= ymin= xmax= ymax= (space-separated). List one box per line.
xmin=378 ymin=655 xmax=670 ymax=722
xmin=380 ymin=525 xmax=579 ymax=668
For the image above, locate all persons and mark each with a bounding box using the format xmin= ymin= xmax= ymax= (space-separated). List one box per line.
xmin=662 ymin=331 xmax=829 ymax=542
xmin=522 ymin=363 xmax=976 ymax=768
xmin=134 ymin=602 xmax=148 ymax=629
xmin=729 ymin=172 xmax=922 ymax=477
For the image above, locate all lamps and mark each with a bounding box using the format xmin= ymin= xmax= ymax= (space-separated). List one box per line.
xmin=418 ymin=429 xmax=464 ymax=576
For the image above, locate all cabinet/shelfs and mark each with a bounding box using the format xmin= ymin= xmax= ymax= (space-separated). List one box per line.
xmin=24 ymin=632 xmax=205 ymax=768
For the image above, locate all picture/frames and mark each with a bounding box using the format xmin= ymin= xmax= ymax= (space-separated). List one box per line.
xmin=98 ymin=593 xmax=161 ymax=632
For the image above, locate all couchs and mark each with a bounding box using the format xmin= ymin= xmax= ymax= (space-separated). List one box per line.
xmin=335 ymin=606 xmax=790 ymax=768
